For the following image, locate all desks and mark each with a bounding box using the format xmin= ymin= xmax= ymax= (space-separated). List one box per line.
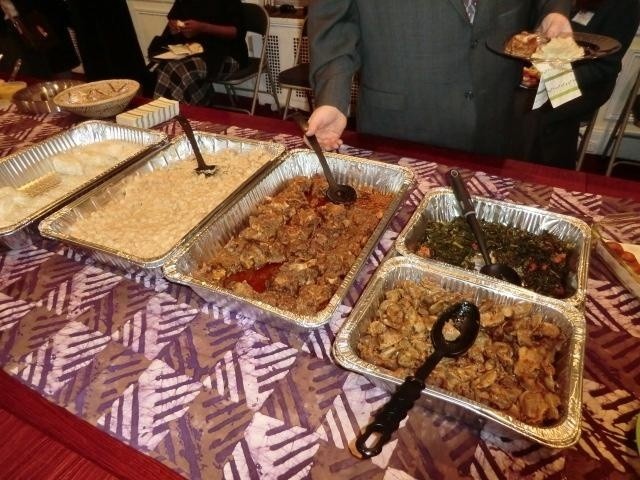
xmin=0 ymin=73 xmax=640 ymax=480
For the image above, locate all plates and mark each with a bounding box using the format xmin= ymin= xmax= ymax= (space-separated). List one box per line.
xmin=485 ymin=32 xmax=623 ymax=64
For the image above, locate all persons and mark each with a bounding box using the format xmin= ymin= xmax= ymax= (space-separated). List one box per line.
xmin=145 ymin=0 xmax=252 ymax=109
xmin=304 ymin=0 xmax=576 ymax=165
xmin=537 ymin=1 xmax=640 ymax=172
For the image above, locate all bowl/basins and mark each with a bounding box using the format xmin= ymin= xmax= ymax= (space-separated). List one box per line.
xmin=53 ymin=78 xmax=141 ymax=119
xmin=13 ymin=78 xmax=87 ymax=115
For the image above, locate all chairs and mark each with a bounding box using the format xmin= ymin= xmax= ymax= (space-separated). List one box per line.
xmin=148 ymin=0 xmax=314 ymax=118
xmin=576 ymin=71 xmax=640 ymax=178
